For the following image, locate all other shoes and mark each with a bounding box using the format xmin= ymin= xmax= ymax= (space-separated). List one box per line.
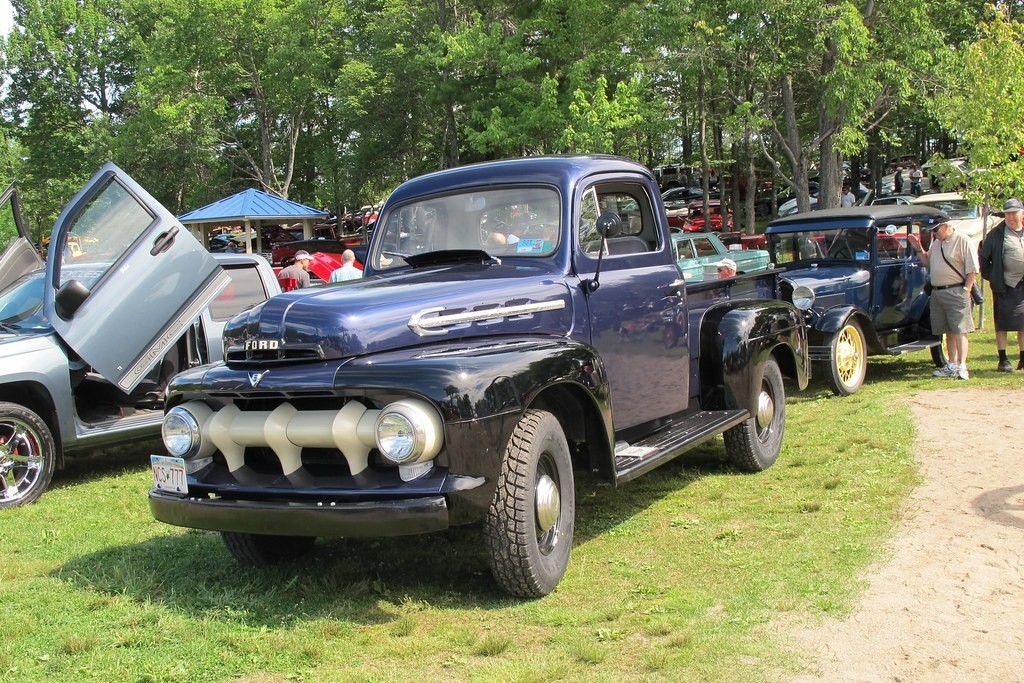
xmin=998 ymin=360 xmax=1013 ymax=372
xmin=1017 ymin=361 xmax=1024 ymax=370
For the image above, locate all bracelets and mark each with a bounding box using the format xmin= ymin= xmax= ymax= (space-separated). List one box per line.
xmin=963 ymin=287 xmax=972 ymax=292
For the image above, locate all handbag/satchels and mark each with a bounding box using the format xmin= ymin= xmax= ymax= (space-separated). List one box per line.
xmin=971 ymin=282 xmax=984 ymax=306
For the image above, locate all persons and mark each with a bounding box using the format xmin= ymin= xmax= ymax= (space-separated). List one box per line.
xmin=277 ymin=250 xmax=314 ymax=292
xmin=907 ymin=212 xmax=979 ymax=380
xmin=713 ymin=259 xmax=737 ymax=278
xmin=507 ymin=226 xmax=523 ymax=244
xmin=895 ymin=167 xmax=904 ymax=193
xmin=909 ymin=166 xmax=923 ymax=195
xmin=978 ymin=199 xmax=1024 ymax=371
xmin=329 ymin=249 xmax=363 ymax=283
xmin=841 ymin=186 xmax=855 ymax=208
xmin=225 ymin=242 xmax=237 ymax=253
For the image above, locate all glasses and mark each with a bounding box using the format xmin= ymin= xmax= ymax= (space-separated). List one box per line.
xmin=843 ymin=190 xmax=847 ymax=191
xmin=933 ymin=223 xmax=944 ymax=232
xmin=718 ymin=268 xmax=728 ymax=274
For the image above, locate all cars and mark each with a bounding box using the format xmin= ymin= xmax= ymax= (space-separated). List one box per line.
xmin=762 ymin=200 xmax=973 ymax=398
xmin=619 ymin=153 xmax=994 ymax=287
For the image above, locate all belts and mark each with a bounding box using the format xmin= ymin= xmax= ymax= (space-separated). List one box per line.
xmin=933 ymin=283 xmax=966 ymax=290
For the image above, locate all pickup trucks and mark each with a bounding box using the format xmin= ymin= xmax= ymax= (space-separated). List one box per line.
xmin=142 ymin=151 xmax=815 ymax=602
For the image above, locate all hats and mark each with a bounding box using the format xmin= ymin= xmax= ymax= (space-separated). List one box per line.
xmin=1002 ymin=198 xmax=1024 ymax=212
xmin=716 ymin=258 xmax=737 ymax=272
xmin=921 ymin=212 xmax=951 ymax=231
xmin=295 ymin=250 xmax=314 ymax=260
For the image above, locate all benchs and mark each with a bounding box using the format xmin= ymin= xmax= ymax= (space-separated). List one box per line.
xmin=878 ymin=249 xmax=916 ymax=259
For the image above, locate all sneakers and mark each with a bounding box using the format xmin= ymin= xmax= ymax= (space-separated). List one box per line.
xmin=952 ymin=367 xmax=968 ymax=380
xmin=933 ymin=364 xmax=958 ymax=378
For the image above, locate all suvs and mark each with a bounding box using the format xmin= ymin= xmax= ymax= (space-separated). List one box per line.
xmin=0 ymin=158 xmax=284 ymax=512
